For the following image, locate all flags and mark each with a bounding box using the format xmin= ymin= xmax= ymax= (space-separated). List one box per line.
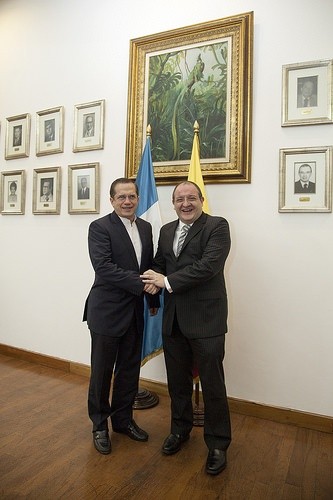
xmin=187 ymin=136 xmax=212 ymax=386
xmin=136 ymin=137 xmax=165 ymax=368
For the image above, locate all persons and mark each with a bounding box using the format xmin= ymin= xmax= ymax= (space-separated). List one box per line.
xmin=40 ymin=179 xmax=52 ymax=202
xmin=79 ymin=178 xmax=89 ymax=199
xmin=13 ymin=127 xmax=21 ymax=146
xmin=294 ymin=164 xmax=315 ymax=193
xmin=44 ymin=122 xmax=55 ymax=142
xmin=136 ymin=180 xmax=231 ymax=476
xmin=83 ymin=117 xmax=94 ymax=137
xmin=8 ymin=182 xmax=17 ymax=203
xmin=297 ymin=81 xmax=316 ymax=107
xmin=81 ymin=176 xmax=160 ymax=456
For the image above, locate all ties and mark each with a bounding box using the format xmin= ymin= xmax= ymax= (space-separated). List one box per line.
xmin=304 ymin=184 xmax=307 ymax=189
xmin=87 ymin=130 xmax=90 ymax=137
xmin=46 ymin=196 xmax=49 ymax=201
xmin=83 ymin=189 xmax=84 ymax=196
xmin=49 ymin=135 xmax=51 ymax=139
xmin=176 ymin=224 xmax=191 ymax=256
xmin=304 ymin=99 xmax=308 ymax=106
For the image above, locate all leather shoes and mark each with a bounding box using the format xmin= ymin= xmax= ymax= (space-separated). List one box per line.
xmin=206 ymin=447 xmax=226 ymax=475
xmin=93 ymin=428 xmax=111 ymax=454
xmin=162 ymin=431 xmax=190 ymax=455
xmin=112 ymin=417 xmax=149 ymax=442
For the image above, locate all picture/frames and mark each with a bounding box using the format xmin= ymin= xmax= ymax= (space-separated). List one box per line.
xmin=279 ymin=145 xmax=333 ymax=213
xmin=0 ymin=170 xmax=26 ymax=215
xmin=72 ymin=99 xmax=106 ymax=153
xmin=67 ymin=161 xmax=101 ymax=215
xmin=281 ymin=58 xmax=333 ymax=128
xmin=4 ymin=112 xmax=32 ymax=161
xmin=31 ymin=166 xmax=62 ymax=216
xmin=125 ymin=10 xmax=255 ymax=186
xmin=36 ymin=105 xmax=65 ymax=157
